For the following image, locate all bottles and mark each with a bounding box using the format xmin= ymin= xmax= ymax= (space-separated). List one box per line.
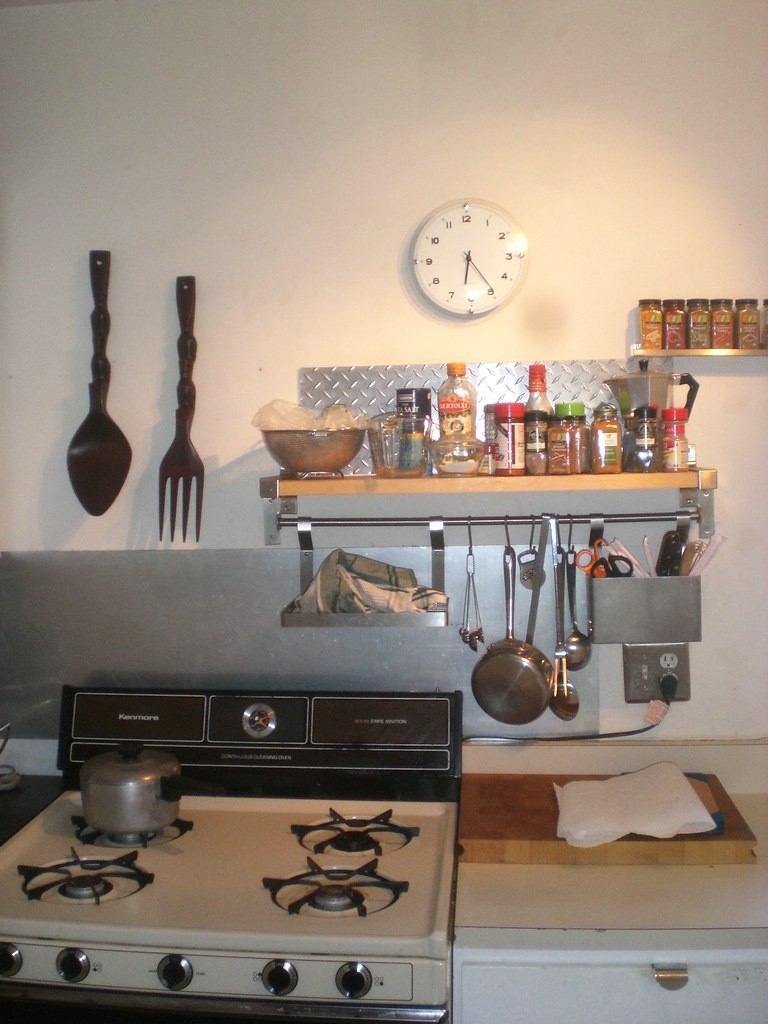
xmin=524 ymin=409 xmax=548 ymax=476
xmin=480 ymin=445 xmax=496 ymax=475
xmin=396 ymin=384 xmax=433 ymax=474
xmin=554 ymin=402 xmax=584 ymax=419
xmin=661 ymin=407 xmax=690 ymax=473
xmin=484 ymin=404 xmax=497 ymax=444
xmin=547 ymin=416 xmax=572 ymax=474
xmin=439 ymin=362 xmax=476 ymax=438
xmin=568 ymin=416 xmax=591 ymax=474
xmin=527 ymin=364 xmax=553 ymax=414
xmin=626 ymin=406 xmax=664 ymax=473
xmin=589 ymin=403 xmax=623 ymax=475
xmin=495 ymin=402 xmax=526 ymax=475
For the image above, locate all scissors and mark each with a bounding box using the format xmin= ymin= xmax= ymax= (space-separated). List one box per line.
xmin=575 ymin=538 xmax=610 ymax=577
xmin=590 ymin=554 xmax=635 ymax=577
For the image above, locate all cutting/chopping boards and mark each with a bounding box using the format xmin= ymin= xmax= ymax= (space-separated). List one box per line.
xmin=460 ymin=773 xmax=758 ymax=865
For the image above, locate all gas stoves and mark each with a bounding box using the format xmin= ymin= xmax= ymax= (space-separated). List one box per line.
xmin=0 ymin=686 xmax=465 ymax=1024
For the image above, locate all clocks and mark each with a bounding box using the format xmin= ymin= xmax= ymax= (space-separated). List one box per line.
xmin=410 ymin=194 xmax=534 ymax=319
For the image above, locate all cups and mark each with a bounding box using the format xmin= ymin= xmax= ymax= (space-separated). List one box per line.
xmin=365 ymin=411 xmax=431 ymax=477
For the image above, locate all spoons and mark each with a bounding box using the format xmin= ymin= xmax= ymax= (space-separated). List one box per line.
xmin=476 ymin=598 xmax=486 ymax=643
xmin=469 ymin=574 xmax=477 ymax=651
xmin=458 ymin=585 xmax=467 ymax=636
xmin=563 ymin=550 xmax=591 ymax=672
xmin=66 ymin=249 xmax=133 ymax=517
xmin=548 ymin=546 xmax=578 ymax=721
xmin=463 ymin=578 xmax=469 ymax=645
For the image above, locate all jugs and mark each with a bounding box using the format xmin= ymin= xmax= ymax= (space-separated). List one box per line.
xmin=602 ymin=360 xmax=699 ymax=470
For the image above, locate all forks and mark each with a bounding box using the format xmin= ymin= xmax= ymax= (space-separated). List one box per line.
xmin=554 ymin=544 xmax=568 ymax=696
xmin=158 ymin=274 xmax=205 ymax=542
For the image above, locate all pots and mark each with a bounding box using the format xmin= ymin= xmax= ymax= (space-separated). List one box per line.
xmin=260 ymin=428 xmax=366 ymax=479
xmin=470 ymin=546 xmax=556 ymax=725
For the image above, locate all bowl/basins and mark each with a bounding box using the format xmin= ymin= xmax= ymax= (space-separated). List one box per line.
xmin=430 ymin=430 xmax=487 ymax=477
